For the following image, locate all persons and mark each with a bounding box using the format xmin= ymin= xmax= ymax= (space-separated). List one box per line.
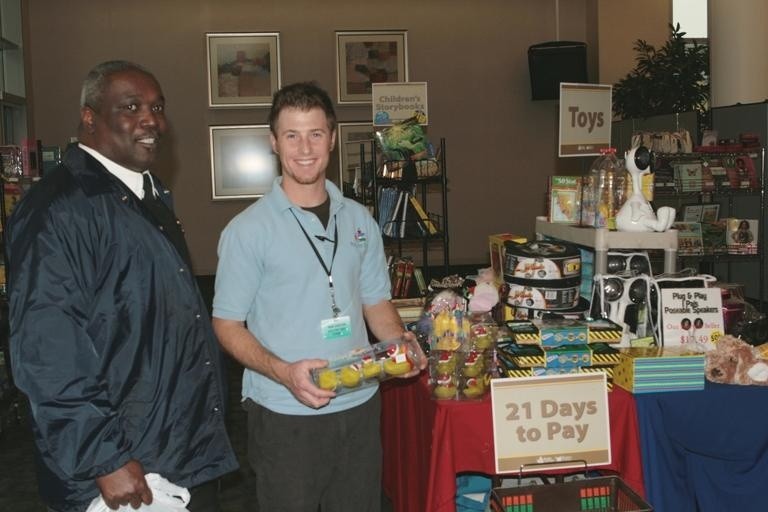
xmin=209 ymin=81 xmax=431 ymax=511
xmin=0 ymin=57 xmax=239 ymax=511
xmin=735 ymin=158 xmax=751 ymax=189
xmin=733 ymin=221 xmax=753 ymax=244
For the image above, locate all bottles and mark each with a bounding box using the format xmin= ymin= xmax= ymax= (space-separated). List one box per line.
xmin=587 ymin=148 xmax=627 ymax=232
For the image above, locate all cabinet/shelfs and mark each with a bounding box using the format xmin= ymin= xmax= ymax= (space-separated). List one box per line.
xmin=534 ymin=215 xmax=680 ymax=278
xmin=649 ymin=145 xmax=768 ymax=347
xmin=360 ymin=137 xmax=450 ymax=299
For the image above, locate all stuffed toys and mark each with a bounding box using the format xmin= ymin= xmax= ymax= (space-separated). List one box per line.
xmin=704 ymin=335 xmax=768 ymax=388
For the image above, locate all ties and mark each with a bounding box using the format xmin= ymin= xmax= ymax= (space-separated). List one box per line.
xmin=141 ymin=174 xmax=155 ymax=196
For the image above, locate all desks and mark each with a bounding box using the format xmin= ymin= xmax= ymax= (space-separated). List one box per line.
xmin=381 ymin=366 xmax=768 ymax=512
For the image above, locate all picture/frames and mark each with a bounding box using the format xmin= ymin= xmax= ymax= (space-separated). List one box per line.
xmin=371 ymin=80 xmax=428 ymax=128
xmin=204 ymin=29 xmax=409 ymax=203
